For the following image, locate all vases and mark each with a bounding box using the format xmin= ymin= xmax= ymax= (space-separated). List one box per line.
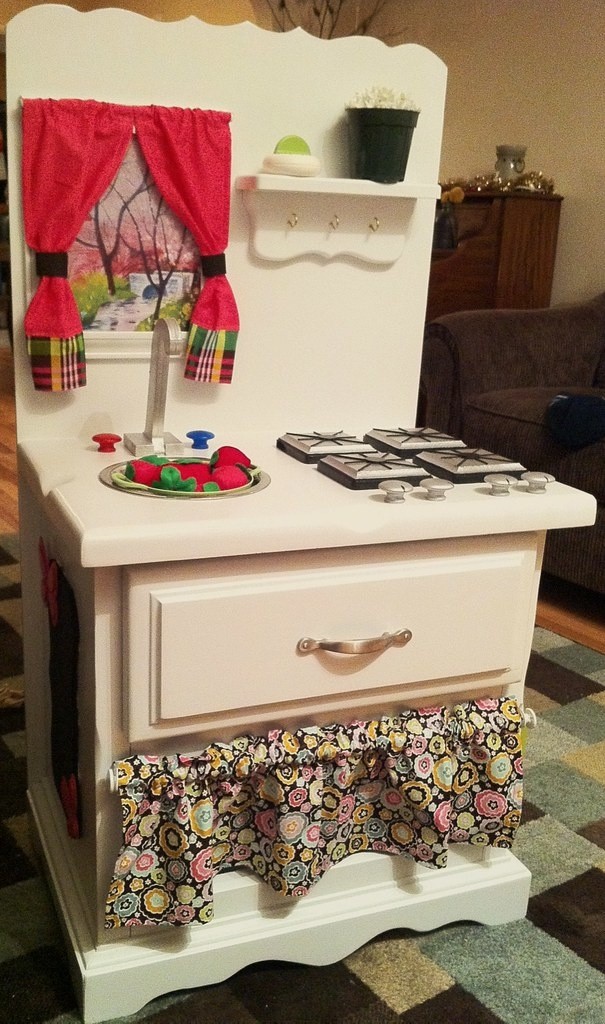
xmin=344 ymin=107 xmax=420 ymax=184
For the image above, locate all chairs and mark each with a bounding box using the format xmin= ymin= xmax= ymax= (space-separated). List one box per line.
xmin=421 ymin=291 xmax=605 ymax=655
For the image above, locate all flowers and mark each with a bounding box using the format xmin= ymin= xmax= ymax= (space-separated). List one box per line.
xmin=345 ymin=86 xmax=422 ymax=113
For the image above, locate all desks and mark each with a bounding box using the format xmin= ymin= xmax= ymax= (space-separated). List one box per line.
xmin=5 ymin=3 xmax=598 ymax=1024
xmin=425 ymin=191 xmax=564 ymax=321
